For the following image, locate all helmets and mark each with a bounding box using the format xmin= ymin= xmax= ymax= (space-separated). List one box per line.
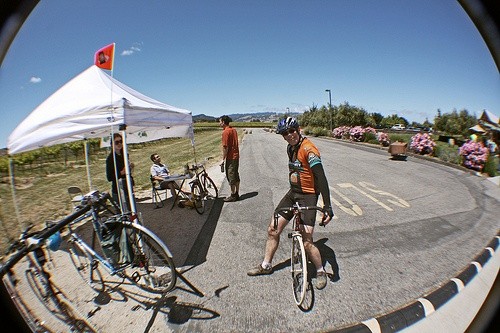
xmin=276 ymin=117 xmax=298 ymax=134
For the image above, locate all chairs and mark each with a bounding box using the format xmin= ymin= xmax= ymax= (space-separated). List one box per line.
xmin=149 ymin=174 xmax=169 ymax=209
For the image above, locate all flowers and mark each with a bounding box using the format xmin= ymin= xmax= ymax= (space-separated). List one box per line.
xmin=332 ymin=124 xmax=491 ymax=174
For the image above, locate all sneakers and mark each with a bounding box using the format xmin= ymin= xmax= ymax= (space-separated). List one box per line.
xmin=223 ymin=196 xmax=239 ymax=201
xmin=247 ymin=264 xmax=273 ymax=276
xmin=316 ymin=269 xmax=327 ymax=290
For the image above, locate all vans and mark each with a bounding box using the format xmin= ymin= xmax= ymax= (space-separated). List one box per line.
xmin=391 ymin=123 xmax=404 ymax=131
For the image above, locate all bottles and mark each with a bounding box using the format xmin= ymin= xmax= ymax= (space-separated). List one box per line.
xmin=221 ymin=163 xmax=224 ymax=172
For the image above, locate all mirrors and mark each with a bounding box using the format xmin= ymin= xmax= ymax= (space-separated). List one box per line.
xmin=0 ymin=0 xmax=500 ymax=333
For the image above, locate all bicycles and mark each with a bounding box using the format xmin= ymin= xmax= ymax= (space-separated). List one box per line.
xmin=188 ymin=156 xmax=218 ymax=214
xmin=273 ymin=203 xmax=325 ymax=305
xmin=1 ymin=225 xmax=100 ymax=333
xmin=67 ymin=192 xmax=176 ymax=293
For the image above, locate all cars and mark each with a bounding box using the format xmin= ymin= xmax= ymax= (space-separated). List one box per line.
xmin=407 ymin=125 xmax=417 ymax=129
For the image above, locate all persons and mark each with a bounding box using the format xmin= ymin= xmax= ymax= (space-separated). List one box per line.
xmin=218 ymin=115 xmax=240 ymax=203
xmin=246 ymin=115 xmax=335 ymax=290
xmin=150 ymin=153 xmax=190 ymax=208
xmin=106 ymin=133 xmax=136 ymax=213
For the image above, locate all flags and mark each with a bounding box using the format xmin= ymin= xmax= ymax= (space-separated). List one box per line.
xmin=94 ymin=43 xmax=114 ymax=70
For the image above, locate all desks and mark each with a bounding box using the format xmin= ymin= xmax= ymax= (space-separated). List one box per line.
xmin=162 ymin=172 xmax=193 ymax=212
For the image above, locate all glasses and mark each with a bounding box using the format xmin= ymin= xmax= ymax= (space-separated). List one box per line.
xmin=115 ymin=140 xmax=123 ymax=145
xmin=283 ymin=129 xmax=297 ymax=136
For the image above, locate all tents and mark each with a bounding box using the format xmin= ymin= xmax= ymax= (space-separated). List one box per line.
xmin=6 ymin=63 xmax=198 ymax=262
